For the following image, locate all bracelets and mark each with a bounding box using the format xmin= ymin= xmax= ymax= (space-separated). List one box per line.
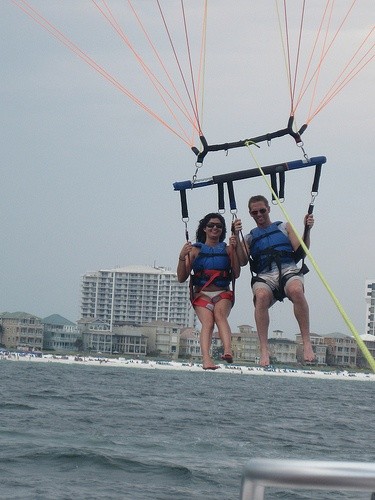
xmin=179 ymin=258 xmax=185 ymax=263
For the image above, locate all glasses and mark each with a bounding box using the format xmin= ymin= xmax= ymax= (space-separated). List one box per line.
xmin=250 ymin=208 xmax=267 ymax=215
xmin=205 ymin=222 xmax=224 ymax=228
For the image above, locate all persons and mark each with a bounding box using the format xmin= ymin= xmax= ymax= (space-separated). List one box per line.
xmin=177 ymin=213 xmax=241 ymax=370
xmin=233 ymin=195 xmax=315 ymax=366
